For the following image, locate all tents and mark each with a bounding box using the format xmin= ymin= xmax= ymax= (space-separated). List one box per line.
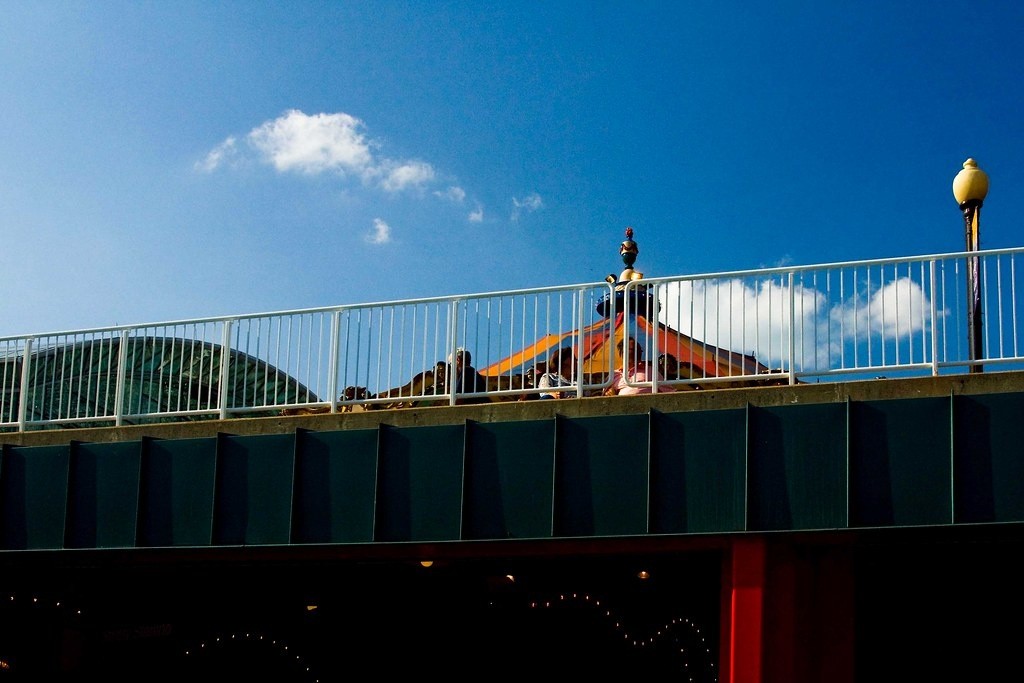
xmin=477 ymin=312 xmax=813 ymax=391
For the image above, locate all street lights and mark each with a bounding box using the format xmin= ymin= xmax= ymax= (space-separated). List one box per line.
xmin=952 ymin=156 xmax=990 ymax=375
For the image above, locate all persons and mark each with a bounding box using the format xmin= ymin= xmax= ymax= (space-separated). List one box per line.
xmin=415 ymin=337 xmax=679 ymax=399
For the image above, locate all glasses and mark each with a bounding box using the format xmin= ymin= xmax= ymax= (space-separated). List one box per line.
xmin=629 ymin=348 xmax=643 ymax=354
